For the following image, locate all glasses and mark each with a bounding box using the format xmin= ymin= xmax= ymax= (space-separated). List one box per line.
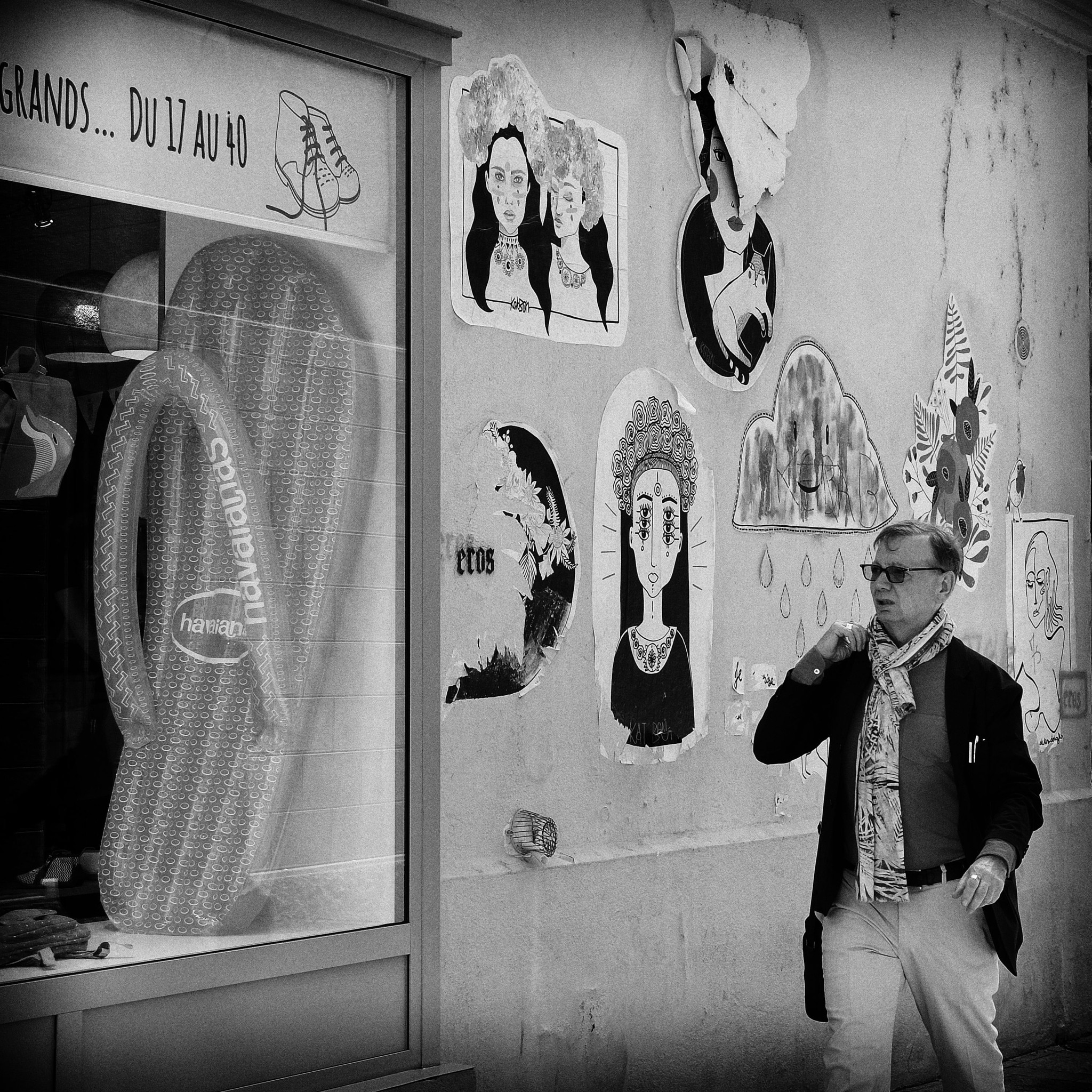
xmin=860 ymin=564 xmax=944 ymax=584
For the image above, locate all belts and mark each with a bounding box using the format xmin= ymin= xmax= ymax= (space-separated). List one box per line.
xmin=846 ymin=857 xmax=966 ymax=887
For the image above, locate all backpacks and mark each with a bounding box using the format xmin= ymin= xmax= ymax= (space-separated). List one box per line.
xmin=0 ymin=909 xmax=91 ymax=965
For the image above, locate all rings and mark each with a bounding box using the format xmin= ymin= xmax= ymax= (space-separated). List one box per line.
xmin=970 ymin=875 xmax=980 ymax=883
xmin=846 ymin=623 xmax=854 ymax=630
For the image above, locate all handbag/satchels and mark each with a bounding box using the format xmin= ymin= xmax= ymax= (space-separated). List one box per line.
xmin=803 ymin=917 xmax=829 ymax=1022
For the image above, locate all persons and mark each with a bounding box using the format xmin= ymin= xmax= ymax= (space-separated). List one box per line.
xmin=752 ymin=520 xmax=1044 ymax=1092
